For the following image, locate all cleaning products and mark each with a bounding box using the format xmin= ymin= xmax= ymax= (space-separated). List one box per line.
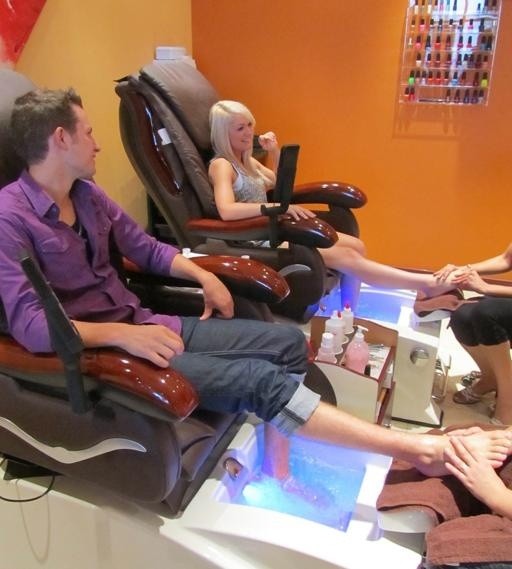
xmin=315 ymin=301 xmax=371 ymax=374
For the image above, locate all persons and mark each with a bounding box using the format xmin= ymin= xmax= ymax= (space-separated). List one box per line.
xmin=1 ymin=86 xmax=511 ymax=507
xmin=434 ymin=244 xmax=511 ymax=427
xmin=443 ymin=426 xmax=512 ymax=519
xmin=207 ymin=98 xmax=472 ymax=317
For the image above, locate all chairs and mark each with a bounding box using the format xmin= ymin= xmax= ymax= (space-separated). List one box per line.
xmin=3 ymin=61 xmax=337 ymax=518
xmin=112 ymin=60 xmax=365 ymax=322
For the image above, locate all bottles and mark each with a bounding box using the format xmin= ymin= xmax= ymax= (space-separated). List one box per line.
xmin=319 ymin=304 xmax=354 ymax=371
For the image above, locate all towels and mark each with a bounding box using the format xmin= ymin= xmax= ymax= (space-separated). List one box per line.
xmin=414 ymin=276 xmax=480 ymax=329
xmin=377 ymin=427 xmax=511 ymax=567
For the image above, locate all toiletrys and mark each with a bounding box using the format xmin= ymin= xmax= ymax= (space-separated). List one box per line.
xmin=402 ymin=1 xmax=498 ymax=104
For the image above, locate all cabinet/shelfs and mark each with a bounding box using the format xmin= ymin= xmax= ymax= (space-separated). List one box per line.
xmin=397 ymin=1 xmax=502 ymax=105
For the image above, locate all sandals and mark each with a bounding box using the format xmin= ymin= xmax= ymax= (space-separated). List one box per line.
xmin=454 ymin=385 xmax=495 ymax=404
